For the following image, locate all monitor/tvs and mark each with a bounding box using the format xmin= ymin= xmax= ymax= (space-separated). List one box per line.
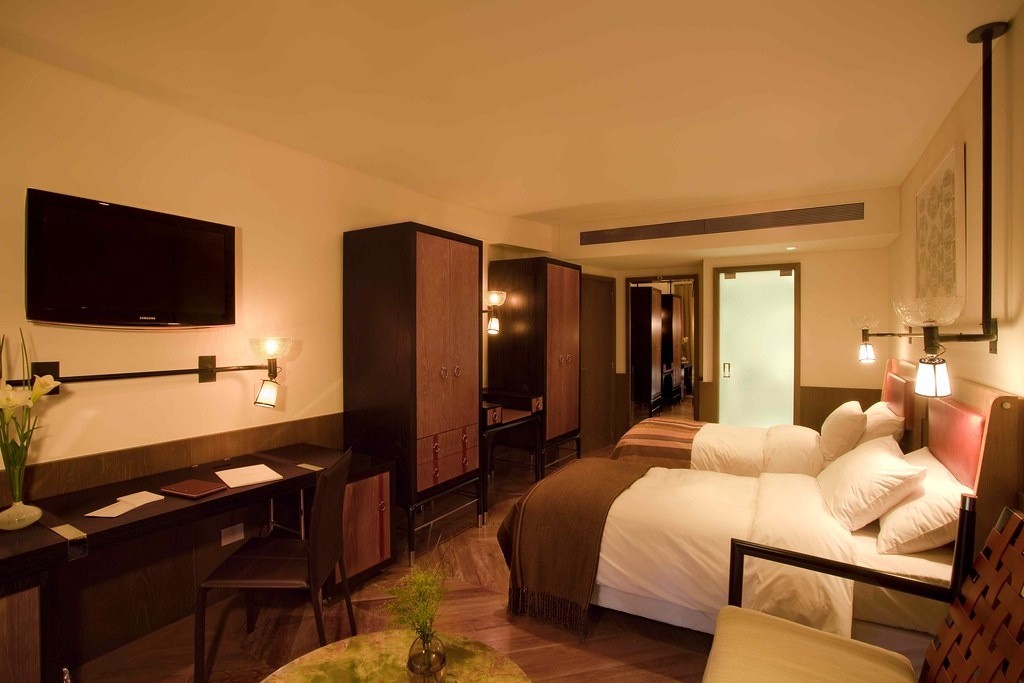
xmin=25 ymin=187 xmax=236 ymax=327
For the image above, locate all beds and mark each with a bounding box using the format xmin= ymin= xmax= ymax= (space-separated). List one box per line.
xmin=497 ymin=357 xmax=1024 ymax=683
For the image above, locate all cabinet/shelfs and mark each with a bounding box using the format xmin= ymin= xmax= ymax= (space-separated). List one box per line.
xmin=661 ymin=294 xmax=682 ymax=411
xmin=487 ymin=256 xmax=581 ymax=481
xmin=630 ymin=286 xmax=662 ymax=418
xmin=341 ymin=220 xmax=484 ymax=570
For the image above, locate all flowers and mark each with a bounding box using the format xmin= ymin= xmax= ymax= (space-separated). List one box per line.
xmin=0 ymin=326 xmax=61 ymax=501
xmin=682 ymin=337 xmax=689 ymax=356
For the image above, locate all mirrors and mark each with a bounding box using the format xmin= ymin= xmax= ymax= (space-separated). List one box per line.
xmin=625 ymin=273 xmax=701 ymax=434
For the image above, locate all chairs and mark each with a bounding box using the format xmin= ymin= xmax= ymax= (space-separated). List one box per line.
xmin=683 ymin=366 xmax=692 ymax=395
xmin=697 ymin=504 xmax=1024 ymax=683
xmin=194 ymin=448 xmax=358 ymax=683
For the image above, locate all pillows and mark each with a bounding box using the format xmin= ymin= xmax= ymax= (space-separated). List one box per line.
xmin=817 ymin=434 xmax=929 ymax=535
xmin=874 ymin=446 xmax=976 ymax=553
xmin=820 ymin=400 xmax=867 ymax=460
xmin=852 ymin=401 xmax=905 ymax=449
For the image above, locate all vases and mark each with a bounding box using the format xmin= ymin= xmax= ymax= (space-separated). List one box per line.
xmin=0 ymin=502 xmax=44 ymax=533
xmin=405 ymin=630 xmax=448 ymax=683
xmin=681 ymin=356 xmax=687 ymax=362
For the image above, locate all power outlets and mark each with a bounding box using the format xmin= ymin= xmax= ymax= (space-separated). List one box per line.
xmin=220 ymin=522 xmax=245 ymax=546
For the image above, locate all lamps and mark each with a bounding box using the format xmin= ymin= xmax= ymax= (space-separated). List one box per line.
xmin=480 ymin=291 xmax=507 ymax=337
xmin=857 ymin=317 xmax=999 ymax=393
xmin=249 ymin=335 xmax=294 ymax=410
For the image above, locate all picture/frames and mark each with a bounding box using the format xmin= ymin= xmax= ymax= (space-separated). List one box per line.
xmin=906 ymin=141 xmax=967 ymax=303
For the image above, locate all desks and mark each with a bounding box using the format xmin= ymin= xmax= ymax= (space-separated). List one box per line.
xmin=0 ymin=441 xmax=396 ymax=683
xmin=482 ymin=407 xmax=542 ymax=512
xmin=259 ymin=629 xmax=535 ymax=683
xmin=662 ymin=368 xmax=673 ymax=412
